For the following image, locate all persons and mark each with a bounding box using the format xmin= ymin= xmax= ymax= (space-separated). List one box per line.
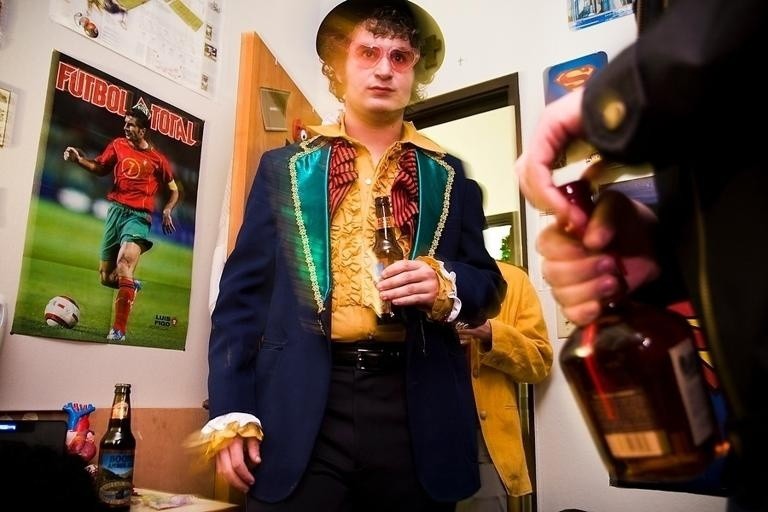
xmin=199 ymin=0 xmax=512 ymax=511
xmin=457 ymin=262 xmax=555 ymax=510
xmin=64 ymin=106 xmax=183 ymax=342
xmin=512 ymin=0 xmax=768 ymax=511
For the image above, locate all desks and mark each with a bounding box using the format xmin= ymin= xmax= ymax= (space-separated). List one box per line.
xmin=131 ymin=487 xmax=238 ymax=512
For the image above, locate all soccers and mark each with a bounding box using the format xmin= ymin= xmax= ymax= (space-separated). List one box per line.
xmin=43 ymin=295 xmax=79 ymax=328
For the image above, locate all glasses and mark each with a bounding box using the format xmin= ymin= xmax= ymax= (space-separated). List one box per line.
xmin=356 ymin=46 xmax=421 ymax=72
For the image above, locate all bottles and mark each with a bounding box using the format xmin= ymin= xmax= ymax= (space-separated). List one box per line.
xmin=373 ymin=195 xmax=406 ymax=326
xmin=96 ymin=384 xmax=136 ymax=509
xmin=556 ymin=179 xmax=716 ymax=489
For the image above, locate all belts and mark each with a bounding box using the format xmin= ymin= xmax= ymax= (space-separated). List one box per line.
xmin=333 ymin=352 xmax=426 ymax=377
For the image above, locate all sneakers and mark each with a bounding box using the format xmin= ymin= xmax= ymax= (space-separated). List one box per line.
xmin=133 ymin=280 xmax=143 ymax=293
xmin=107 ymin=327 xmax=126 ymax=343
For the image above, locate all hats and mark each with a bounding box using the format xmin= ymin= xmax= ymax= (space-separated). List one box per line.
xmin=316 ymin=0 xmax=445 ymax=80
